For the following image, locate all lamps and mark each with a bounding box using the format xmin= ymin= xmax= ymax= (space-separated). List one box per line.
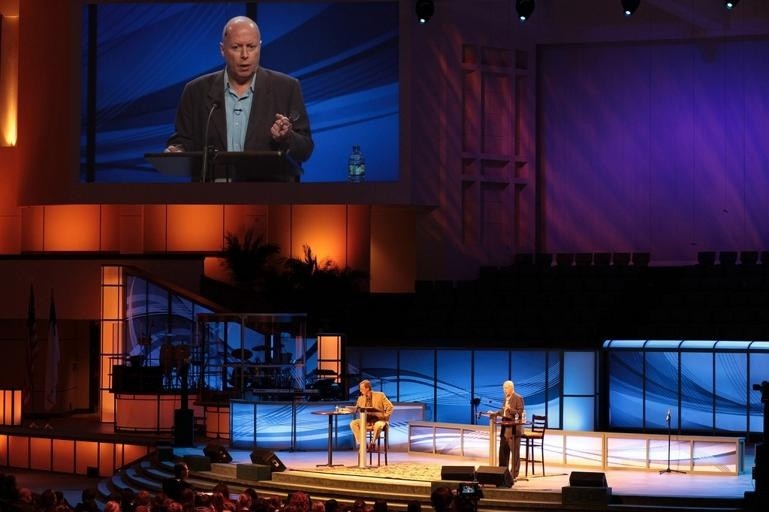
xmin=522 ymin=414 xmax=547 ymax=478
xmin=358 ymin=410 xmax=388 ymax=467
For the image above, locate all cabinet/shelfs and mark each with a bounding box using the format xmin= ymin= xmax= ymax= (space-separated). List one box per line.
xmin=522 ymin=410 xmax=527 ymax=422
xmin=347 ymin=146 xmax=366 ymax=183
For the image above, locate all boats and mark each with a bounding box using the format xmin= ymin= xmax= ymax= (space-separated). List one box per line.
xmin=569 ymin=471 xmax=607 ymax=487
xmin=441 ymin=466 xmax=474 ymax=483
xmin=696 ymin=250 xmax=769 ymax=265
xmin=513 ymin=251 xmax=652 ymax=267
xmin=475 ymin=466 xmax=514 ymax=488
xmin=249 ymin=449 xmax=286 ymax=472
xmin=203 ymin=444 xmax=234 ymax=464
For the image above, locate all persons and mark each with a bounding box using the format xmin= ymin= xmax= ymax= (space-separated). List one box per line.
xmin=334 ymin=379 xmax=394 ymax=453
xmin=486 ymin=380 xmax=525 ymax=482
xmin=165 ymin=16 xmax=314 ymax=182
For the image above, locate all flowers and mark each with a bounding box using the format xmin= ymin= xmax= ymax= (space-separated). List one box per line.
xmin=200 ymin=101 xmax=219 ymax=182
xmin=666 ymin=411 xmax=671 ymax=422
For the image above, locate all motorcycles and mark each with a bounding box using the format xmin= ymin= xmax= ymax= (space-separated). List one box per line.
xmin=660 ymin=424 xmax=688 ymax=474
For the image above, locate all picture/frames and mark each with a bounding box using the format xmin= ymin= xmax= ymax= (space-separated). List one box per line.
xmin=310 ymin=409 xmax=352 ymax=466
xmin=495 ymin=420 xmax=529 ymax=481
